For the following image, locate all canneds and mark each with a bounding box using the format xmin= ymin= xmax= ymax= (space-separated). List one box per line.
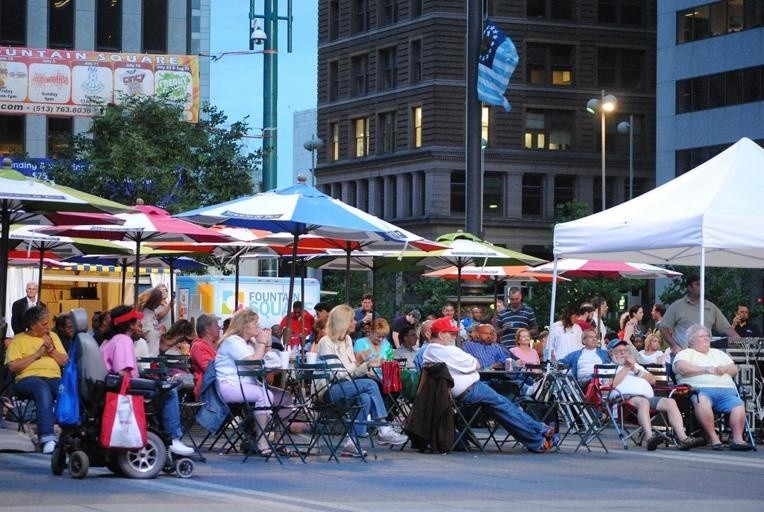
xmin=505 ymin=357 xmax=513 ymax=372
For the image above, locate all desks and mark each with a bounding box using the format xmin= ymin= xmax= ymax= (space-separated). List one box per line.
xmin=442 ymin=369 xmax=567 ymax=451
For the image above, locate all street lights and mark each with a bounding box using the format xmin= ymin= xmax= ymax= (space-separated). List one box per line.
xmin=250 ymin=12 xmax=276 ymax=191
xmin=617 ymin=115 xmax=637 ymax=202
xmin=586 ymin=88 xmax=618 ymax=211
xmin=302 ymin=133 xmax=322 ymax=188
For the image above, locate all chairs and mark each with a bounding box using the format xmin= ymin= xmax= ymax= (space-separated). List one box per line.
xmin=292 ymin=363 xmax=369 ymax=465
xmin=2 ymin=351 xmax=86 ymax=434
xmin=182 ymin=373 xmax=273 ymax=455
xmin=137 ymin=358 xmax=207 ymax=461
xmin=553 ymin=365 xmax=618 ymax=451
xmin=423 ymin=361 xmax=484 ymax=453
xmin=319 ymin=354 xmax=389 ymax=460
xmin=235 ymin=359 xmax=310 ymax=465
xmin=500 ymin=363 xmax=571 ymax=453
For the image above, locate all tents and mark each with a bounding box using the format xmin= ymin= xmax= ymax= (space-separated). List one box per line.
xmin=548 ymin=136 xmax=764 ymax=358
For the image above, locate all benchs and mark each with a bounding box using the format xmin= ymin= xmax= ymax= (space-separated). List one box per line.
xmin=584 ymin=364 xmax=757 ymax=452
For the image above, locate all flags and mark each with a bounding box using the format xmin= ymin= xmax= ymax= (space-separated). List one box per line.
xmin=476 ymin=18 xmax=519 ymax=111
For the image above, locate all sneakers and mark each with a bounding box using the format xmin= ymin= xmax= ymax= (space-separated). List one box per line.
xmin=42 ymin=441 xmax=57 ymax=454
xmin=341 ymin=444 xmax=369 ymax=457
xmin=676 ymin=436 xmax=705 ymax=451
xmin=528 ymin=433 xmax=561 ymax=453
xmin=377 ymin=430 xmax=409 ymax=445
xmin=647 ymin=432 xmax=666 ymax=451
xmin=168 ymin=439 xmax=194 ymax=457
xmin=541 ymin=426 xmax=555 ymax=439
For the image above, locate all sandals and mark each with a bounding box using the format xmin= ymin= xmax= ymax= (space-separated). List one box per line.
xmin=254 ymin=447 xmax=280 ymax=458
xmin=276 ymin=446 xmax=300 ymax=457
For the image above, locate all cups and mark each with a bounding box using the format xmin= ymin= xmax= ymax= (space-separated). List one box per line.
xmin=306 ymin=352 xmax=316 ymax=365
xmin=367 ymin=312 xmax=372 ymax=322
xmin=656 ymin=355 xmax=664 ymax=363
xmin=281 ymin=352 xmax=290 ymax=369
xmin=385 ymin=345 xmax=393 ymax=361
xmin=181 ymin=343 xmax=191 ymax=355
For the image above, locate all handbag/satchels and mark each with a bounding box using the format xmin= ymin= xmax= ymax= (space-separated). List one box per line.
xmin=99 ymin=391 xmax=148 ymax=450
xmin=54 ymin=362 xmax=81 ymax=425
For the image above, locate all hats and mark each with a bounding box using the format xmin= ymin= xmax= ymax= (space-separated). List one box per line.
xmin=410 ymin=309 xmax=422 ymax=320
xmin=607 ymin=338 xmax=628 ymax=350
xmin=431 ymin=318 xmax=460 ymax=333
xmin=111 ymin=307 xmax=145 ymax=324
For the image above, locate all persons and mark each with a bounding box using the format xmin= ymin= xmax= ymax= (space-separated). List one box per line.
xmin=5 ymin=279 xmax=763 ymax=459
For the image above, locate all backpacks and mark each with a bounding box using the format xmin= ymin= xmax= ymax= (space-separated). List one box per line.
xmin=584 ymin=382 xmax=601 ymax=405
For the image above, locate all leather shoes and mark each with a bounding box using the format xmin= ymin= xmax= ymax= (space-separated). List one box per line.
xmin=729 ymin=442 xmax=755 ymax=452
xmin=712 ymin=443 xmax=725 ymax=450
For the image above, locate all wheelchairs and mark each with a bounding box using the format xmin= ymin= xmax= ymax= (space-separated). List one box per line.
xmin=51 ymin=307 xmax=194 ymax=481
